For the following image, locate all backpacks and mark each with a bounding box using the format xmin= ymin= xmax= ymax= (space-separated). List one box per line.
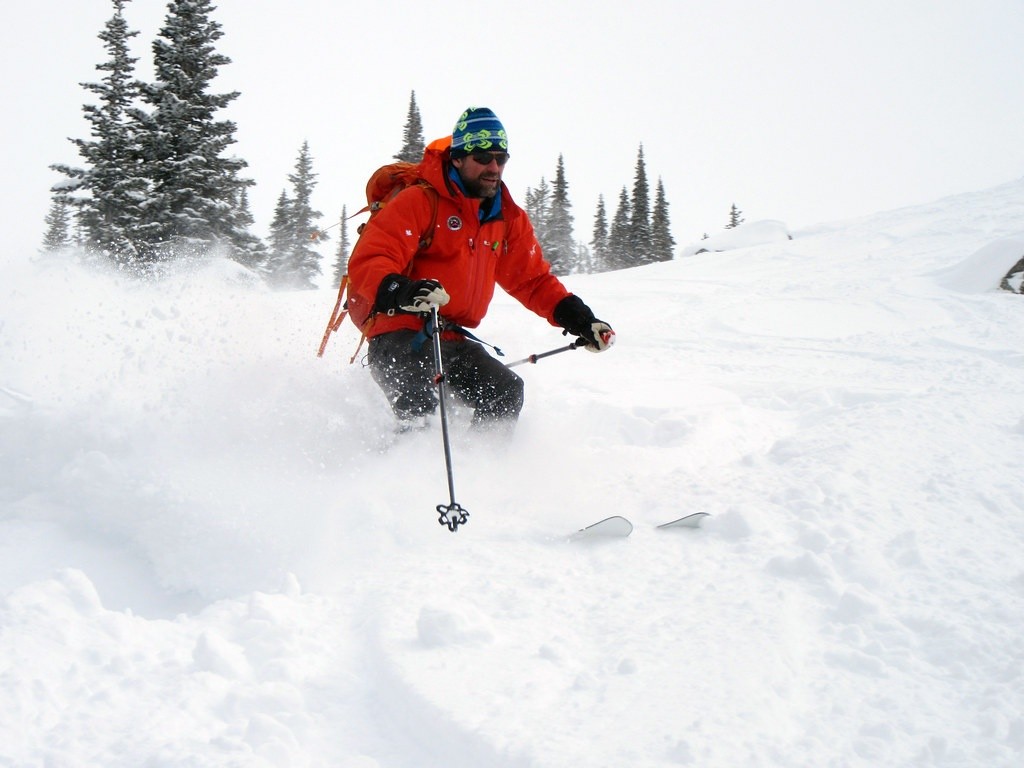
xmin=330 ymin=164 xmax=508 ymax=335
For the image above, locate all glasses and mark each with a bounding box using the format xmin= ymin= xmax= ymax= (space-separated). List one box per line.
xmin=467 ymin=152 xmax=510 ymax=165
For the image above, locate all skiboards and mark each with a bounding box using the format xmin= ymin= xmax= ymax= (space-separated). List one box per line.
xmin=566 ymin=510 xmax=711 ymax=538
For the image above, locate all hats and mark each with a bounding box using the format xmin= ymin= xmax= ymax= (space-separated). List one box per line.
xmin=450 ymin=108 xmax=508 ymax=158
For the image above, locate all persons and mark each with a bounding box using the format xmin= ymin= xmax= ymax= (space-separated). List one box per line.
xmin=346 ymin=107 xmax=616 ymax=434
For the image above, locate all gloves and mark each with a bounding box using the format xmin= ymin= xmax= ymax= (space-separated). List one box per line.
xmin=554 ymin=296 xmax=616 ymax=353
xmin=375 ymin=273 xmax=450 ymax=319
